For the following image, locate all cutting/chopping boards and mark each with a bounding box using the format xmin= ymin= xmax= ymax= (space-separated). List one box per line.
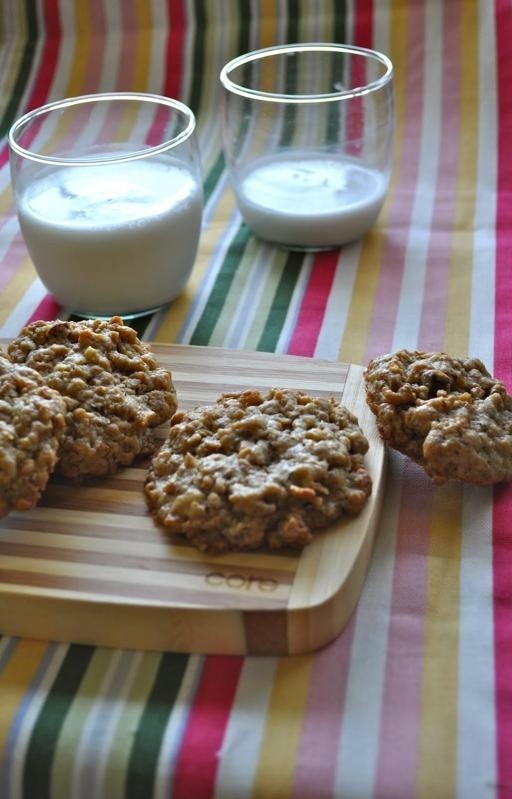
xmin=3 ymin=338 xmax=390 ymax=659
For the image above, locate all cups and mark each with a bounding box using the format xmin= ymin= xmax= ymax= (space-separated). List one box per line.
xmin=6 ymin=87 xmax=205 ymax=323
xmin=216 ymin=36 xmax=397 ymax=256
